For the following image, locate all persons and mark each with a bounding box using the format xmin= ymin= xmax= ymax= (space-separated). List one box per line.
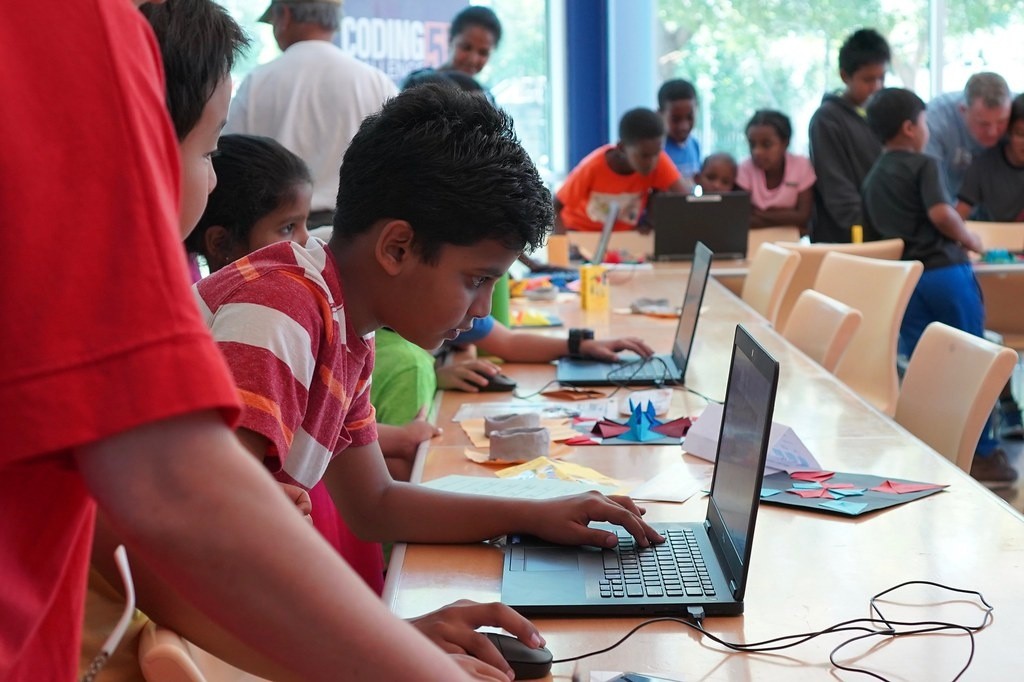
xmin=77 ymin=1 xmax=510 ymax=682
xmin=549 ymin=107 xmax=685 ymax=236
xmin=192 ymin=83 xmax=667 ymax=682
xmin=732 ymin=109 xmax=816 ymax=242
xmin=658 ymin=79 xmax=704 ymax=196
xmin=221 ymin=1 xmax=401 ymax=244
xmin=957 ymin=94 xmax=1024 ymax=265
xmin=183 ymin=133 xmax=386 ymax=596
xmin=923 ymin=72 xmax=1020 ymax=221
xmin=691 ymin=151 xmax=738 ymax=194
xmin=0 ymin=0 xmax=481 ymax=682
xmin=439 ymin=5 xmax=502 ymax=78
xmin=370 ymin=66 xmax=654 ymax=581
xmin=859 ymin=87 xmax=1024 ymax=489
xmin=810 ymin=28 xmax=884 ymax=244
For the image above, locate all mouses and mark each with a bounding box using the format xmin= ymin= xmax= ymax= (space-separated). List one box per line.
xmin=462 ymin=368 xmax=517 ymax=392
xmin=467 ymin=632 xmax=553 ymax=679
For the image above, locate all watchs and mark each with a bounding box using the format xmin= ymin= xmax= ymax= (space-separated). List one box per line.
xmin=568 ymin=329 xmax=594 ymax=359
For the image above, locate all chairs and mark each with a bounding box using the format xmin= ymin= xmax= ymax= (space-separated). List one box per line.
xmin=743 ymin=221 xmax=1024 ymax=474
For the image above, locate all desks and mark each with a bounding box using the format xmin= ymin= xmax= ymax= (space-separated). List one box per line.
xmin=382 ymin=275 xmax=1024 ymax=681
xmin=566 ymin=212 xmax=1024 ymax=303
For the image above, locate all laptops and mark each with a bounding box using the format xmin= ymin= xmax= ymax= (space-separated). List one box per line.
xmin=556 ymin=242 xmax=714 ymax=386
xmin=591 ymin=202 xmax=622 ymax=264
xmin=645 ymin=189 xmax=753 ymax=261
xmin=501 ymin=324 xmax=780 ymax=618
